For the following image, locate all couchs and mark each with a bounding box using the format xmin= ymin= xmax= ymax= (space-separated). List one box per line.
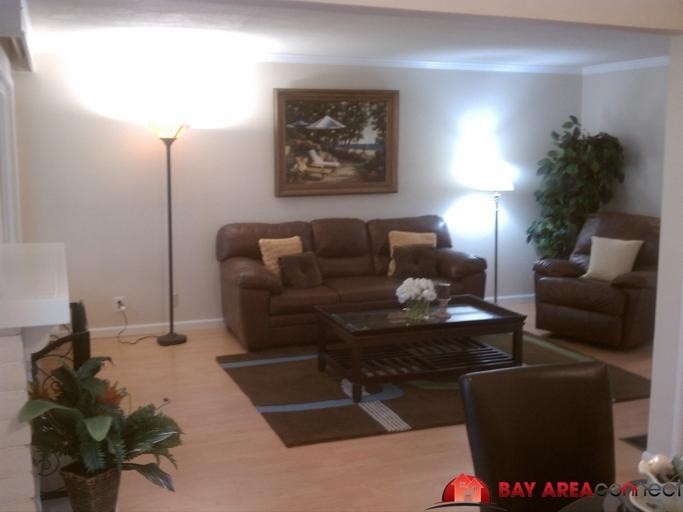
xmin=531 ymin=212 xmax=659 ymax=349
xmin=215 ymin=214 xmax=489 ymax=352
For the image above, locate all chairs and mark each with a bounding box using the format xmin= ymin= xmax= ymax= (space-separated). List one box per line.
xmin=458 ymin=360 xmax=616 ymax=511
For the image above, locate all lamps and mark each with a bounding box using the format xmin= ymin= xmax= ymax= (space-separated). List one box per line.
xmin=148 ymin=111 xmax=190 ymax=349
xmin=473 ymin=164 xmax=517 ymax=307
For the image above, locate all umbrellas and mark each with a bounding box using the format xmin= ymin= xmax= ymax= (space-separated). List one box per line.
xmin=305 ymin=114 xmax=349 ymax=149
xmin=293 ymin=118 xmax=307 ymax=127
xmin=285 ymin=124 xmax=294 ymax=128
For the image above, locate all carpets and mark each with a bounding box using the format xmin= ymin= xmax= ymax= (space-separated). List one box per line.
xmin=214 ymin=327 xmax=652 ymax=450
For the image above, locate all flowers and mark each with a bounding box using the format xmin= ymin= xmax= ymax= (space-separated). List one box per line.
xmin=394 ymin=276 xmax=438 ymax=305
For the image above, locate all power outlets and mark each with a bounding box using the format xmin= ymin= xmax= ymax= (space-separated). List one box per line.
xmin=112 ymin=296 xmax=127 ymax=313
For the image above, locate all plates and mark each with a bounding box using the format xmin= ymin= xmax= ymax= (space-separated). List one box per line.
xmin=628 ymin=483 xmax=682 ymax=512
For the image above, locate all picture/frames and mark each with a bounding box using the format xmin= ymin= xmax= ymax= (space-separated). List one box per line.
xmin=272 ymin=87 xmax=400 ymax=196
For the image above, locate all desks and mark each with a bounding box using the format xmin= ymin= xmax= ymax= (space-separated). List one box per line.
xmin=559 ymin=474 xmax=682 ymax=511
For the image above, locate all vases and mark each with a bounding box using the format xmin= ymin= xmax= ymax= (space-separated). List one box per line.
xmin=402 ymin=301 xmax=430 ymax=319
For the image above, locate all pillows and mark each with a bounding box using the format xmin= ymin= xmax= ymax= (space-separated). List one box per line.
xmin=386 ymin=228 xmax=436 ymax=278
xmin=257 ymin=237 xmax=305 ymax=275
xmin=578 ymin=236 xmax=644 ymax=288
xmin=395 ymin=244 xmax=436 ymax=281
xmin=278 ymin=253 xmax=323 ymax=289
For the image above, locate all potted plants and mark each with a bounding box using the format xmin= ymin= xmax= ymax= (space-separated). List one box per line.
xmin=13 ymin=349 xmax=185 ymax=512
